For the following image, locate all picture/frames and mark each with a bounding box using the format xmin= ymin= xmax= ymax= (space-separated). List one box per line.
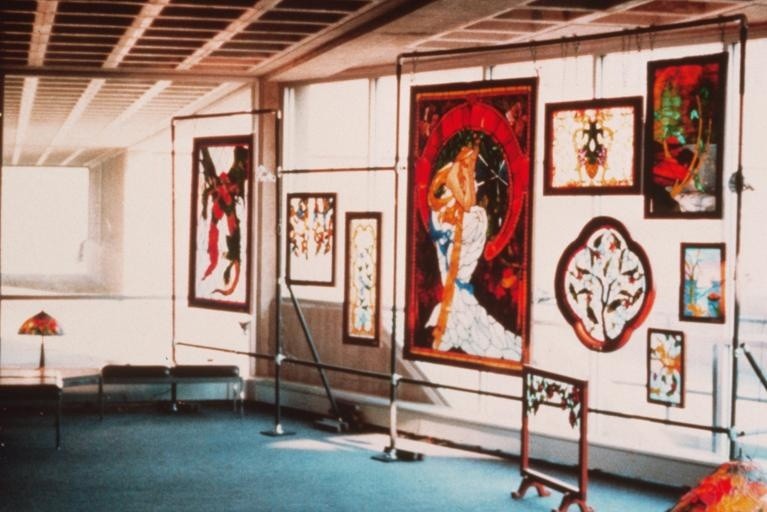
xmin=679 ymin=242 xmax=725 ymax=323
xmin=285 ymin=192 xmax=336 ymax=285
xmin=189 ymin=134 xmax=254 ymax=311
xmin=555 ymin=217 xmax=655 ymax=351
xmin=643 ymin=53 xmax=727 ymax=219
xmin=545 ymin=95 xmax=644 ymax=196
xmin=342 ymin=212 xmax=380 ymax=346
xmin=404 ymin=77 xmax=537 ymax=376
xmin=647 ymin=327 xmax=685 ymax=406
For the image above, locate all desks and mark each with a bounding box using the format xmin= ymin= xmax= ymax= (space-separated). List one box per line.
xmin=1 ymin=367 xmax=104 ymax=451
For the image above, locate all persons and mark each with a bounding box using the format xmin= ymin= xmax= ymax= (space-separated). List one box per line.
xmin=425 ymin=143 xmax=521 ymax=361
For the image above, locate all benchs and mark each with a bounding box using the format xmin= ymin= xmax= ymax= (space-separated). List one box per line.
xmin=104 ymin=365 xmax=245 ymax=415
